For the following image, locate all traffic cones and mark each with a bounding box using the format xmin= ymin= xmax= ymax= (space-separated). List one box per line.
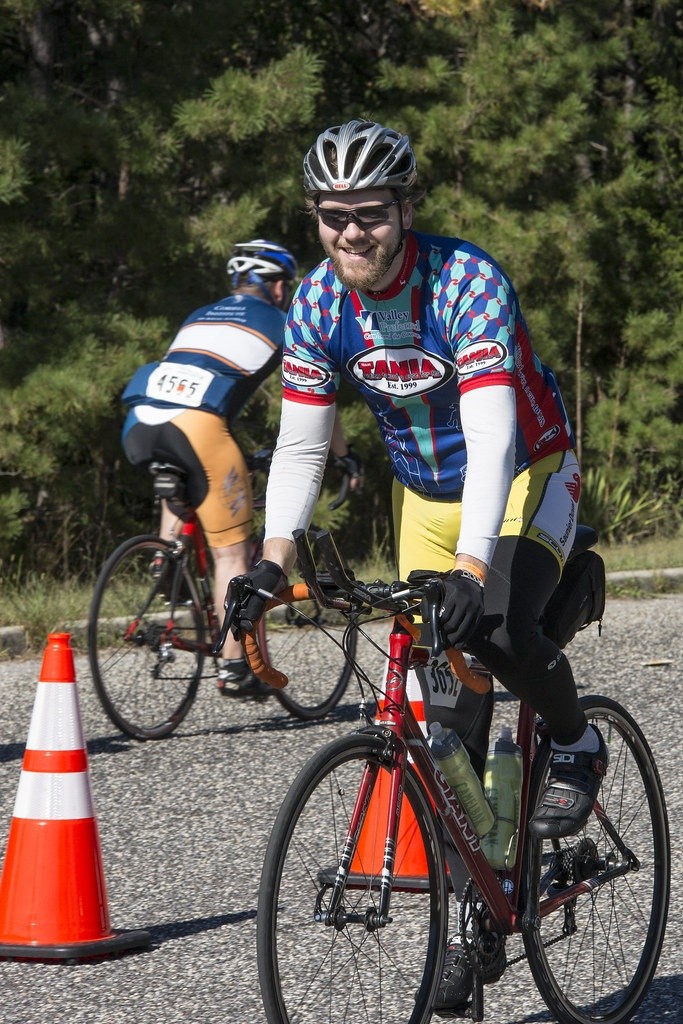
xmin=314 ymin=640 xmax=456 ymax=892
xmin=0 ymin=631 xmax=151 ymax=962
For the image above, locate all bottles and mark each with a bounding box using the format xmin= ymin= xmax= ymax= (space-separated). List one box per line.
xmin=479 ymin=727 xmax=523 ymax=871
xmin=429 ymin=722 xmax=494 ymax=837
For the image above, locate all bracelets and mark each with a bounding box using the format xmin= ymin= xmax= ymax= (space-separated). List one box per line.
xmin=451 ymin=562 xmax=485 ymax=584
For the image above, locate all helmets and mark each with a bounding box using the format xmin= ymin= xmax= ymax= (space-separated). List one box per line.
xmin=302 ymin=121 xmax=418 ymax=197
xmin=226 ymin=239 xmax=298 ymax=282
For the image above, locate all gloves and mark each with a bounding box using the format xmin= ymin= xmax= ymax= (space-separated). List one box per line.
xmin=223 ymin=559 xmax=289 ymax=641
xmin=421 ymin=569 xmax=485 ymax=651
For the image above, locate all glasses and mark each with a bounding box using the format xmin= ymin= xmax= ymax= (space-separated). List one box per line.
xmin=313 ymin=193 xmax=404 ymax=231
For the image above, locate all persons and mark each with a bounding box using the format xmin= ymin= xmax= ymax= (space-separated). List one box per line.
xmin=124 ymin=238 xmax=359 ymax=699
xmin=227 ymin=119 xmax=608 ymax=1013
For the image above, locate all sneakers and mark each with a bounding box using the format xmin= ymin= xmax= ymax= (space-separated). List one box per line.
xmin=415 ymin=930 xmax=507 ymax=1008
xmin=217 ymin=669 xmax=279 ymax=698
xmin=149 ymin=550 xmax=193 ymax=604
xmin=527 ymin=723 xmax=609 ymax=839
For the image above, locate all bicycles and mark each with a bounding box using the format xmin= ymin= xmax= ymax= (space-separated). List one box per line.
xmin=209 ymin=528 xmax=673 ymax=1024
xmin=84 ymin=441 xmax=361 ymax=744
